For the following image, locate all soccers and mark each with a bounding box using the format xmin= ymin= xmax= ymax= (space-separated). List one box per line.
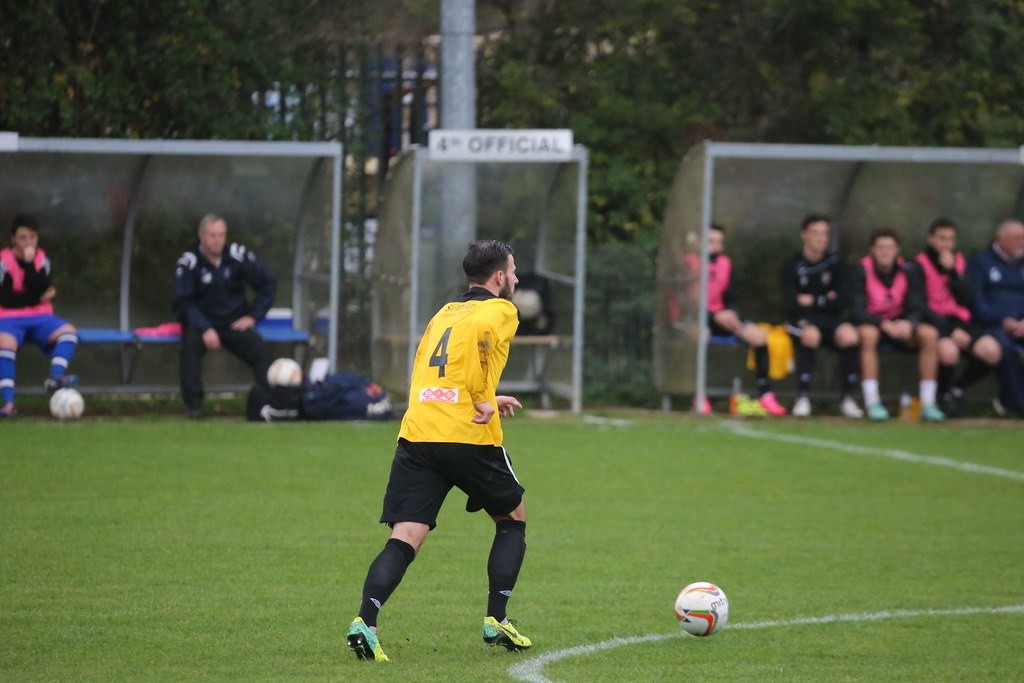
xmin=672 ymin=580 xmax=729 ymax=638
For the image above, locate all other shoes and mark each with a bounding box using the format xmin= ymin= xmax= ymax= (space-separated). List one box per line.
xmin=0 ymin=402 xmax=17 ymax=416
xmin=993 ymin=391 xmax=1013 ymax=418
xmin=694 ymin=395 xmax=712 ymax=416
xmin=791 ymin=397 xmax=812 ymax=417
xmin=46 ymin=375 xmax=80 ymax=390
xmin=921 ymin=402 xmax=946 ymax=421
xmin=842 ymin=397 xmax=864 ymax=418
xmin=864 ymin=402 xmax=890 ymax=421
xmin=185 ymin=393 xmax=203 ymax=418
xmin=759 ymin=391 xmax=787 ymax=417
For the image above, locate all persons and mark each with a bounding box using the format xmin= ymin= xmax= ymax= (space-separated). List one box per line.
xmin=968 ymin=216 xmax=1024 ymax=418
xmin=849 ymin=227 xmax=946 ymax=422
xmin=913 ymin=216 xmax=1003 ymax=414
xmin=0 ymin=215 xmax=80 ymax=419
xmin=663 ymin=221 xmax=788 ymax=416
xmin=782 ymin=213 xmax=866 ymax=418
xmin=347 ymin=239 xmax=533 ymax=661
xmin=173 ymin=212 xmax=278 ymax=421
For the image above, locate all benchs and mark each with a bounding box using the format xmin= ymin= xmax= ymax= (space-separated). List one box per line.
xmin=70 ymin=329 xmax=310 ymax=389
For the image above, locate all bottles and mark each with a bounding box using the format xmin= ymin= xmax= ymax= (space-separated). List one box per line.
xmin=899 ymin=388 xmax=913 ymax=424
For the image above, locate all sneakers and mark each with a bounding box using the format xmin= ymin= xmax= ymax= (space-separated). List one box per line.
xmin=483 ymin=616 xmax=532 ymax=651
xmin=347 ymin=617 xmax=388 ymax=662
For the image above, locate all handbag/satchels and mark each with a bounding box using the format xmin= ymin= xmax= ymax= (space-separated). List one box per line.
xmin=243 ymin=359 xmax=396 ymax=423
